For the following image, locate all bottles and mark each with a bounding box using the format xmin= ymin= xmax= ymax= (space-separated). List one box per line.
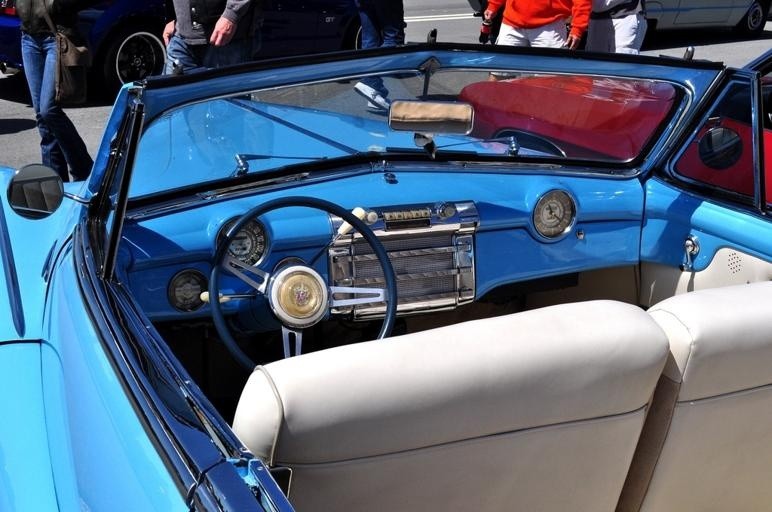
xmin=478 ymin=15 xmax=493 ymax=44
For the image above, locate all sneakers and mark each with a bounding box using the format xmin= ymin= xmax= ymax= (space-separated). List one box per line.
xmin=353 ymin=81 xmax=390 ymax=111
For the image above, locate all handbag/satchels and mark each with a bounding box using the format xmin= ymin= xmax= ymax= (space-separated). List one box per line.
xmin=53 ymin=28 xmax=94 ymax=110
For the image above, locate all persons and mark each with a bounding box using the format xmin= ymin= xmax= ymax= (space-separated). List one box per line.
xmin=585 ymin=0 xmax=648 ymax=56
xmin=355 ymin=0 xmax=408 ymax=113
xmin=483 ymin=0 xmax=593 ymax=84
xmin=154 ymin=0 xmax=249 ymax=75
xmin=1 ymin=1 xmax=95 ymax=182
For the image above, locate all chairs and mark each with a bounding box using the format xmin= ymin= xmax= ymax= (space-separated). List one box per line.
xmin=232 ymin=298 xmax=676 ymax=512
xmin=612 ymin=276 xmax=772 ymax=511
